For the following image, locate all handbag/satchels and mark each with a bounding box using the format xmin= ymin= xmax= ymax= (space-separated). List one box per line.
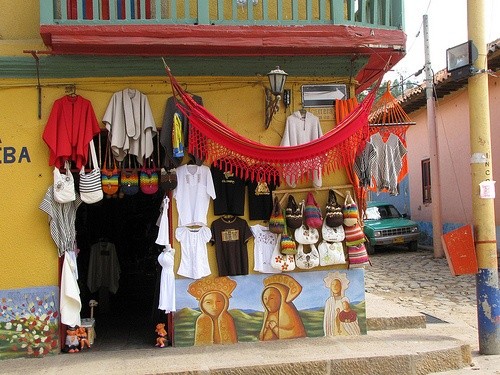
xmin=269 ymin=191 xmax=369 ymax=272
xmin=53 ymin=138 xmax=177 ymax=204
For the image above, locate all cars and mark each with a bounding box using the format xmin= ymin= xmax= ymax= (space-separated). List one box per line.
xmin=361 ymin=201 xmax=421 ymax=256
